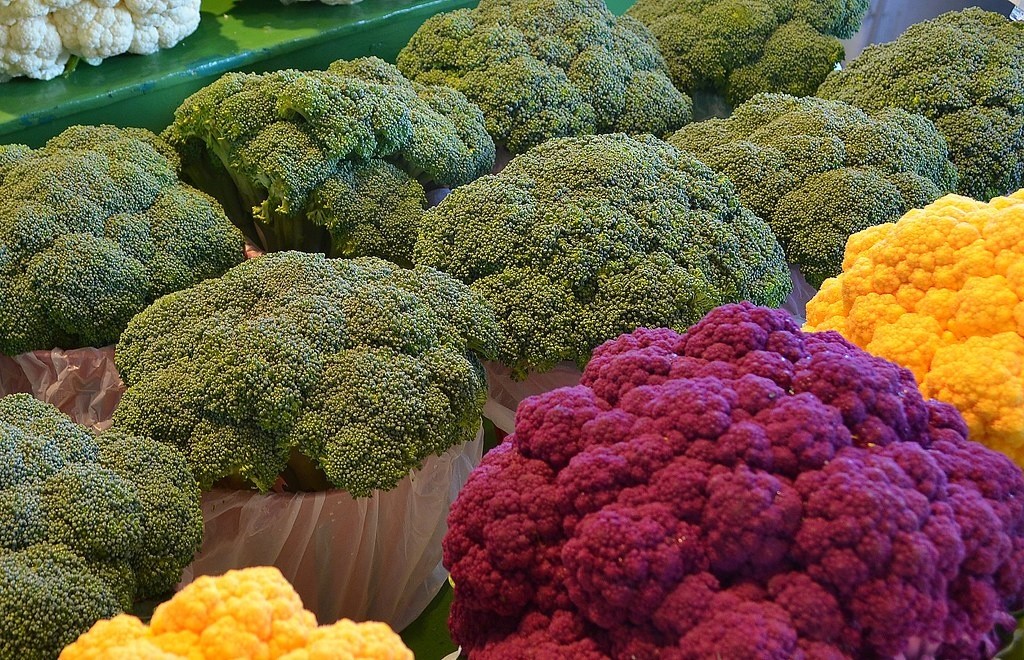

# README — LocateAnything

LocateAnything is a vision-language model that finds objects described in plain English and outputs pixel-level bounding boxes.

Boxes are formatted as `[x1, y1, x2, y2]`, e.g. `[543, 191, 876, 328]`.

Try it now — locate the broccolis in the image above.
[0, 0, 1024, 660]
